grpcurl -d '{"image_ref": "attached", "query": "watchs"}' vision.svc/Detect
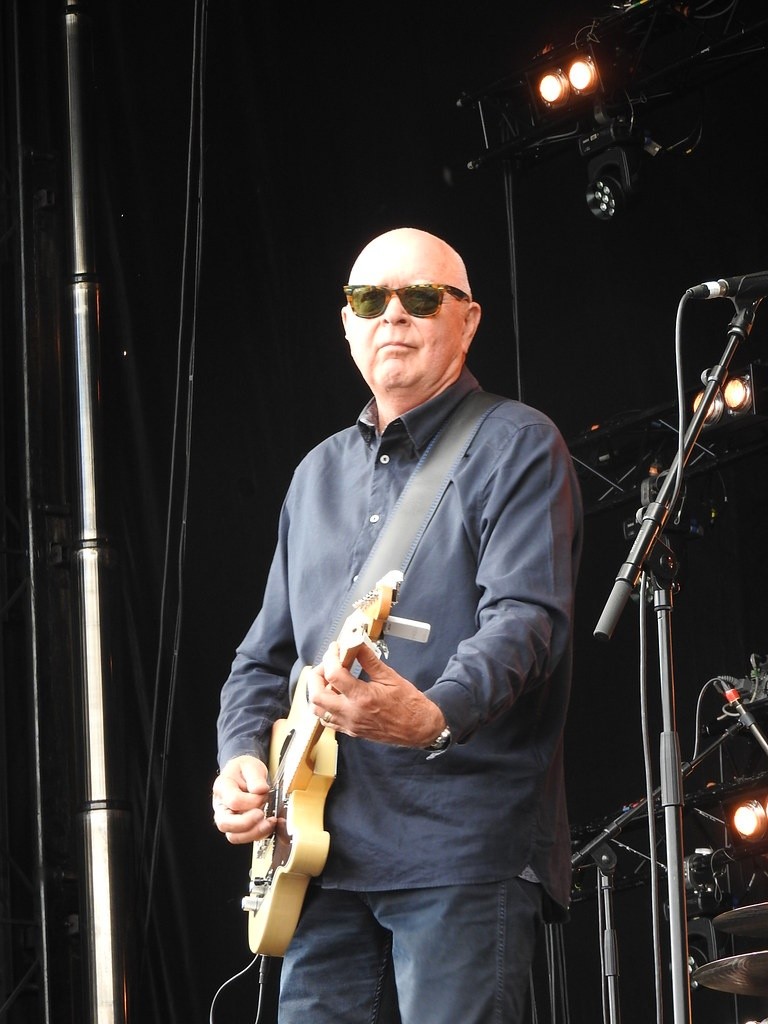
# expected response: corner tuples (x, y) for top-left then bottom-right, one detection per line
(424, 722), (453, 754)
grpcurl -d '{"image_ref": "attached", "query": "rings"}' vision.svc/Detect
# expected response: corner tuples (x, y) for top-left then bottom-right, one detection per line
(322, 710), (333, 723)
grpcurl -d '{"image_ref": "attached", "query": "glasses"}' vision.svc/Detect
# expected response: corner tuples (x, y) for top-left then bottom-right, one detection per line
(343, 285), (472, 319)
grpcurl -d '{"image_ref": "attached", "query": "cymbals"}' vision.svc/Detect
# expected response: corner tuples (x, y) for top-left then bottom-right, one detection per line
(710, 900), (768, 940)
(692, 950), (768, 1001)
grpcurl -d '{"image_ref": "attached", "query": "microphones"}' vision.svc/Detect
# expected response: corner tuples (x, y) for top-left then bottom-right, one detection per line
(686, 270), (768, 304)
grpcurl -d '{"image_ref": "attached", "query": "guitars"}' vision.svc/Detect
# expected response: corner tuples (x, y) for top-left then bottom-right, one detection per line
(238, 571), (403, 958)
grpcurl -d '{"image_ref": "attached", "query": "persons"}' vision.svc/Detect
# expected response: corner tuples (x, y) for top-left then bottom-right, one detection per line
(209, 227), (580, 1024)
